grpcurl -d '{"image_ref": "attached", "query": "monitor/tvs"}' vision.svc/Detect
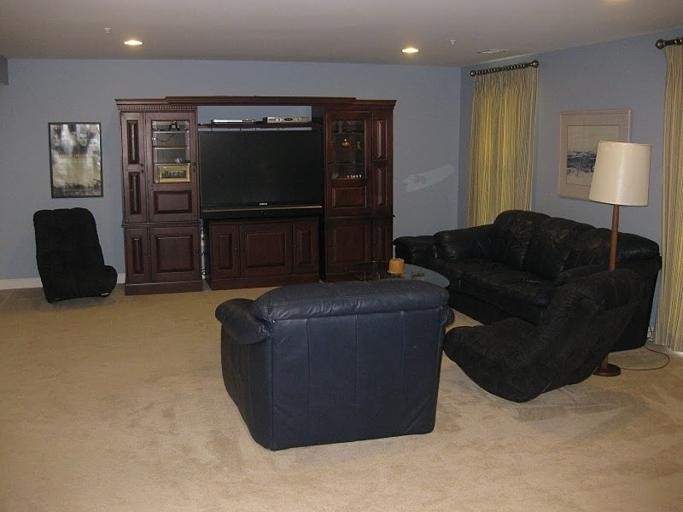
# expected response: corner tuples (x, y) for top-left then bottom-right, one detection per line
(198, 124), (324, 219)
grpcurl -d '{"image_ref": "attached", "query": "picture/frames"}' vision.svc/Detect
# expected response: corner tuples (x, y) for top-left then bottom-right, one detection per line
(556, 106), (634, 203)
(47, 120), (106, 200)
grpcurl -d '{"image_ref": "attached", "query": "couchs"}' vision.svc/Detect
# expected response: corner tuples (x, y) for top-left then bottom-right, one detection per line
(392, 207), (665, 354)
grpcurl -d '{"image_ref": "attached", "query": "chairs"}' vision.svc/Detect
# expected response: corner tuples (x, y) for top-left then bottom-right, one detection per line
(211, 276), (456, 454)
(32, 206), (119, 304)
(443, 264), (650, 403)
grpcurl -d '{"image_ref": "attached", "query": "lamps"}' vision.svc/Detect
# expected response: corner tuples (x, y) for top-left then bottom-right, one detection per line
(588, 139), (653, 378)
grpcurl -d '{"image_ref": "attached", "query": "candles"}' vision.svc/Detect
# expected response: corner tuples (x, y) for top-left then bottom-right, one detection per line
(387, 256), (405, 272)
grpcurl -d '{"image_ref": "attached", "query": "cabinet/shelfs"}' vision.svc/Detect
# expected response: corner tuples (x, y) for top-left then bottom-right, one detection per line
(203, 218), (323, 292)
(311, 96), (399, 282)
(112, 98), (202, 296)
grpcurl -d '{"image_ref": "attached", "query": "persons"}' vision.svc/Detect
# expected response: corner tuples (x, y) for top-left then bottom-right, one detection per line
(50, 123), (100, 164)
